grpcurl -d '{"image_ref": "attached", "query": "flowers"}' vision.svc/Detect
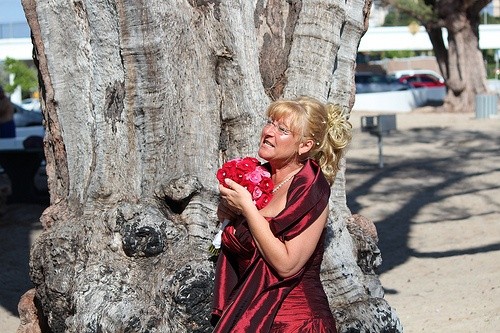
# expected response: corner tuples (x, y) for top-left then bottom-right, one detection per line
(207, 156), (274, 257)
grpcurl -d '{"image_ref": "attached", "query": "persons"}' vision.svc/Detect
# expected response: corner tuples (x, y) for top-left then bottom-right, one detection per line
(212, 96), (353, 333)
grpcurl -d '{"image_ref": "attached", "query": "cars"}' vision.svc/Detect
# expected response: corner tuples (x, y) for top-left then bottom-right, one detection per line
(21, 98), (41, 113)
(0, 99), (47, 149)
(399, 74), (447, 88)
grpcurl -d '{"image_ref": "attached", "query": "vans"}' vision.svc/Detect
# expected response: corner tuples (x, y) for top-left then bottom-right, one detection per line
(395, 69), (445, 84)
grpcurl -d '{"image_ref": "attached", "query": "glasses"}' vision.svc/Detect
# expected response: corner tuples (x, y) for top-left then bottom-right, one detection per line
(264, 118), (308, 138)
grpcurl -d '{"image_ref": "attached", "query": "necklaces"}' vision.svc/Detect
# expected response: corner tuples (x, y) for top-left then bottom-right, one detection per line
(272, 165), (304, 192)
